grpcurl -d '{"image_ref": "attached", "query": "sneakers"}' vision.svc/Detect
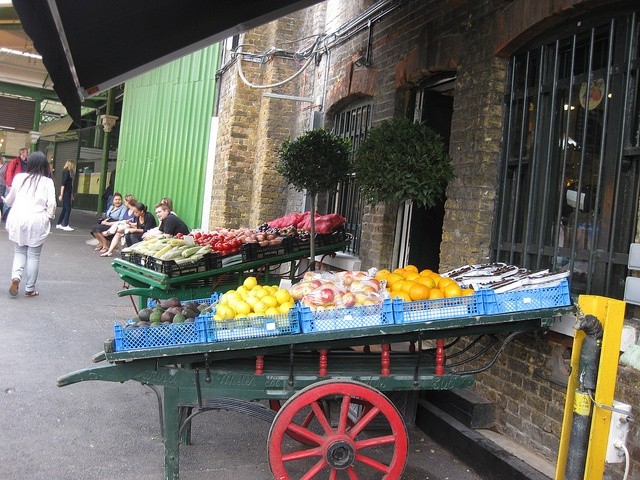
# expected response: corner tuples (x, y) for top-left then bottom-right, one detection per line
(24, 291), (39, 296)
(62, 226), (74, 231)
(9, 278), (20, 296)
(56, 224), (63, 229)
(101, 252), (112, 257)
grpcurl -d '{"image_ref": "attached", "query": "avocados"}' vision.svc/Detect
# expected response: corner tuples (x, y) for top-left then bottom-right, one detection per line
(127, 297), (209, 333)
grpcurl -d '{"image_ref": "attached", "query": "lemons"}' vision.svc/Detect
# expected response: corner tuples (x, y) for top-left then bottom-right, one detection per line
(213, 277), (294, 330)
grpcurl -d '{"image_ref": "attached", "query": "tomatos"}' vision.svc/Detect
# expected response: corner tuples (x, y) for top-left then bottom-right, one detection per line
(176, 231), (243, 257)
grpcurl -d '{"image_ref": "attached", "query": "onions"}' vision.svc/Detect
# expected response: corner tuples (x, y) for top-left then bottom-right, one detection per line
(254, 222), (317, 240)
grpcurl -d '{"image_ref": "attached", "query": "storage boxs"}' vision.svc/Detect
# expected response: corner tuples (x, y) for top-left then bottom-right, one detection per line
(484, 277), (571, 315)
(113, 293), (218, 351)
(205, 302), (300, 345)
(394, 290), (483, 326)
(301, 296), (393, 333)
(121, 221), (346, 277)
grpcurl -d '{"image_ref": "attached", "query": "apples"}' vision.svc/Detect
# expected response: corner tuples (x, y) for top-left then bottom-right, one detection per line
(293, 268), (388, 326)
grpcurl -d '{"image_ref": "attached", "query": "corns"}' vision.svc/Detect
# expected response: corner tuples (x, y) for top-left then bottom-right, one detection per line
(122, 234), (211, 272)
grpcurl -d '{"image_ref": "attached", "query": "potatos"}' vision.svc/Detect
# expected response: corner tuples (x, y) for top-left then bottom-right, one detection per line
(213, 228), (283, 246)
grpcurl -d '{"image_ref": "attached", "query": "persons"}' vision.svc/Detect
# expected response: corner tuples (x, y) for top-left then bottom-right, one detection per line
(156, 198), (177, 228)
(0, 153), (8, 213)
(102, 169), (115, 218)
(154, 203), (189, 235)
(100, 194), (138, 257)
(56, 159), (76, 231)
(90, 192), (128, 253)
(122, 203), (157, 247)
(0, 150), (57, 297)
(1, 148), (28, 224)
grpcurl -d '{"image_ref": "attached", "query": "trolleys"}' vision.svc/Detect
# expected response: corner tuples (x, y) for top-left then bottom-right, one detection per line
(285, 406), (289, 410)
(115, 229), (351, 301)
(58, 304), (579, 480)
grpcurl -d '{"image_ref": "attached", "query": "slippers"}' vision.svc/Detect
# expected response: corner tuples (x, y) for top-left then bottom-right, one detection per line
(100, 248), (109, 252)
(95, 245), (103, 250)
